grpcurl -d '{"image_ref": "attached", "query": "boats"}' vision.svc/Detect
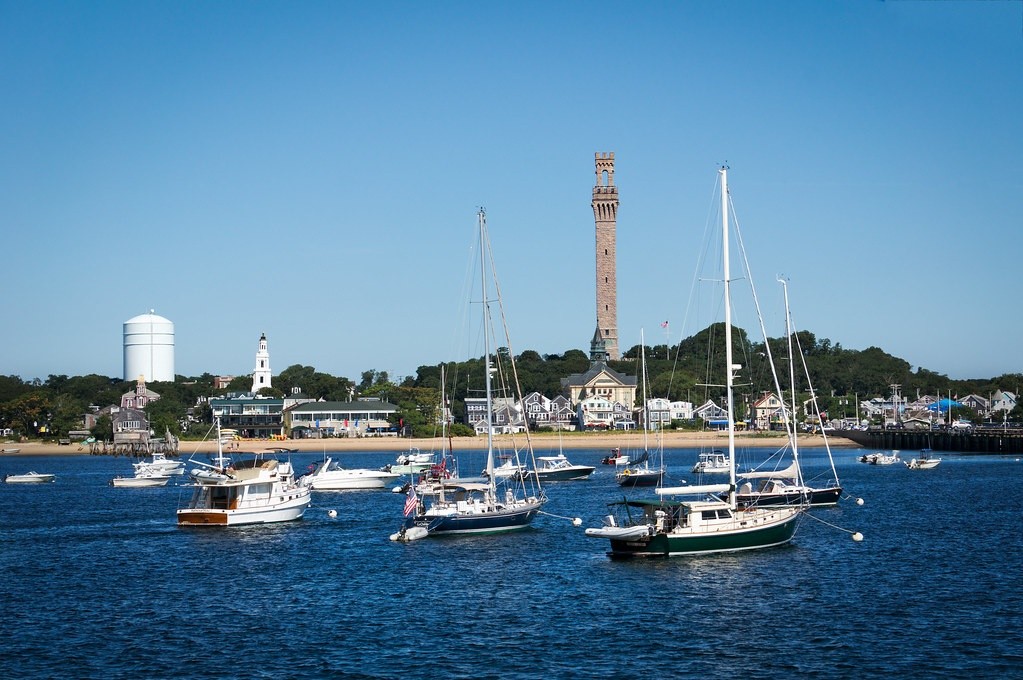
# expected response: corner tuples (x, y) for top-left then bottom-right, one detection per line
(295, 441), (402, 491)
(903, 435), (943, 471)
(132, 451), (187, 476)
(5, 471), (59, 484)
(1, 448), (22, 453)
(174, 415), (312, 530)
(108, 459), (172, 488)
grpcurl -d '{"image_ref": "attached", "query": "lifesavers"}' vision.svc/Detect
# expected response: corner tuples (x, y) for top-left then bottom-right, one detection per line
(624, 470), (629, 474)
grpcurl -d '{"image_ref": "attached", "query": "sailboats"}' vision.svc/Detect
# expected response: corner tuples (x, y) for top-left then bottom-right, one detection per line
(377, 158), (901, 562)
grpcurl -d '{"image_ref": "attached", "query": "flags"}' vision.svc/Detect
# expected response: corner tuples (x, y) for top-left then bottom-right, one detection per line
(404, 488), (418, 516)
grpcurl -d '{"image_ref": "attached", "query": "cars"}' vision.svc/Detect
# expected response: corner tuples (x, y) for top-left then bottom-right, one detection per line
(951, 420), (973, 431)
(0, 428), (13, 437)
(806, 425), (820, 433)
(819, 424), (835, 432)
(866, 422), (886, 435)
(886, 421), (904, 431)
